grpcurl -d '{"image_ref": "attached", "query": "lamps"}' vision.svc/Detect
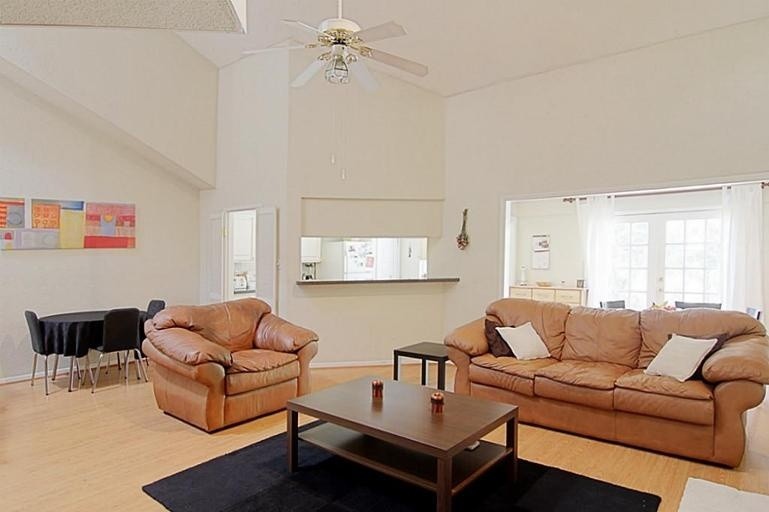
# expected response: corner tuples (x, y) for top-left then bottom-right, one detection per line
(322, 45), (357, 88)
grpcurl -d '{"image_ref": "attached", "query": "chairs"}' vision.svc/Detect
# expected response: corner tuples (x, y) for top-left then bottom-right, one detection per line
(23, 310), (94, 392)
(83, 307), (149, 393)
(598, 299), (762, 321)
(125, 300), (167, 383)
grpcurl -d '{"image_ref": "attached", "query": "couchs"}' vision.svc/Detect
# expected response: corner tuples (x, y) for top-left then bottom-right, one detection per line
(446, 298), (767, 477)
(142, 296), (319, 432)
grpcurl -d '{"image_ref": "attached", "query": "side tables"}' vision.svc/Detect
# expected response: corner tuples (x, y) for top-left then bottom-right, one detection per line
(392, 340), (449, 389)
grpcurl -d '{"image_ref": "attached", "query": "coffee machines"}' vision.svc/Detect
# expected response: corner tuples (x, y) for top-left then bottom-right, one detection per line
(301, 262), (317, 281)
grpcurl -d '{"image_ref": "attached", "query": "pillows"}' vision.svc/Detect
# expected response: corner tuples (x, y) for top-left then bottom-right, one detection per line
(483, 319), (511, 357)
(495, 321), (553, 362)
(667, 333), (728, 379)
(643, 332), (719, 385)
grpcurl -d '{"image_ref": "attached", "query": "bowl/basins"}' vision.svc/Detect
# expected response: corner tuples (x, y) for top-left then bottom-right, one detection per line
(537, 282), (552, 286)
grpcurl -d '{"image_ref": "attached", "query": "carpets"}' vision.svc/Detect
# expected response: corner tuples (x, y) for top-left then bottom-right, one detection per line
(677, 477), (768, 512)
(142, 421), (664, 512)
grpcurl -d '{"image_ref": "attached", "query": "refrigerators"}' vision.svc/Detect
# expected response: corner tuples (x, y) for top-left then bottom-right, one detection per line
(322, 237), (378, 281)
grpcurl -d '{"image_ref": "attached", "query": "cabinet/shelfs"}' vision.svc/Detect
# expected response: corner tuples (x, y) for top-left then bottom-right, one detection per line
(230, 211), (256, 265)
(300, 235), (322, 263)
(508, 286), (590, 305)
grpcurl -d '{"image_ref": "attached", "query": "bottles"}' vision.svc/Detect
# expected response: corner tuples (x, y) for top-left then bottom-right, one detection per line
(430, 390), (444, 414)
(371, 379), (384, 398)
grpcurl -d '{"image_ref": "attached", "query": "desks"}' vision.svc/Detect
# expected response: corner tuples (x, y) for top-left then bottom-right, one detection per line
(38, 309), (146, 393)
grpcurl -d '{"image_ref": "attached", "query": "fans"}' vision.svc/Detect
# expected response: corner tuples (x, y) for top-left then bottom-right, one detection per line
(243, 0), (430, 89)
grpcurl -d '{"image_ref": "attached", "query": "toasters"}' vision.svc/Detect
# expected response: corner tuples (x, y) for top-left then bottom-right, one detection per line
(234, 272), (249, 289)
